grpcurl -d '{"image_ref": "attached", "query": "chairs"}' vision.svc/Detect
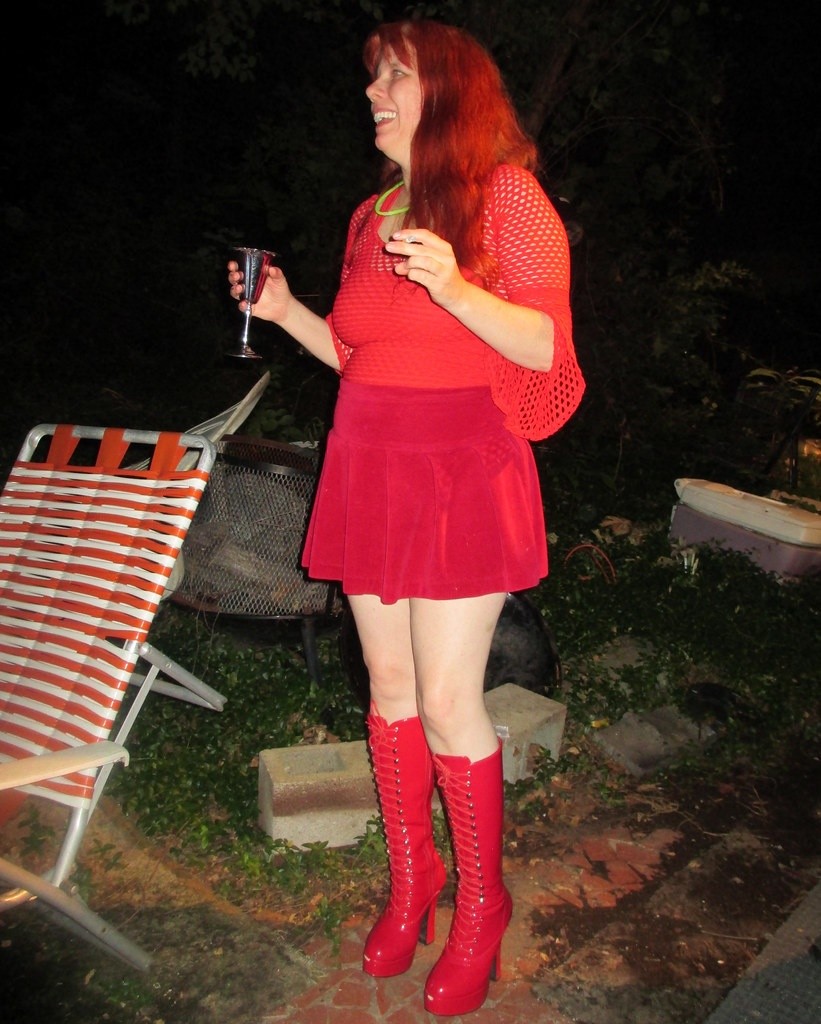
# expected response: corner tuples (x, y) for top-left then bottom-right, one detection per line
(0, 420), (227, 981)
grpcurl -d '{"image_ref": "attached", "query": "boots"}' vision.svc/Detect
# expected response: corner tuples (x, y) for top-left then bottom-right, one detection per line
(423, 735), (513, 1017)
(362, 714), (447, 977)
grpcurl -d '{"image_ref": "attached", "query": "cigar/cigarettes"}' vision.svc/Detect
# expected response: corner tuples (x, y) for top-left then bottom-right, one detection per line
(402, 237), (415, 244)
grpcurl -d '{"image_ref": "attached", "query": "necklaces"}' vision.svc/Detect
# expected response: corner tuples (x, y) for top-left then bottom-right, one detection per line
(374, 181), (428, 216)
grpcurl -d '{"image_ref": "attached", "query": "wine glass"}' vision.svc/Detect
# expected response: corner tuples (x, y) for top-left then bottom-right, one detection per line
(226, 246), (276, 360)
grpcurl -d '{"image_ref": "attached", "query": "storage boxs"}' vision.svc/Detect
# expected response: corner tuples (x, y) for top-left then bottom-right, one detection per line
(668, 478), (821, 584)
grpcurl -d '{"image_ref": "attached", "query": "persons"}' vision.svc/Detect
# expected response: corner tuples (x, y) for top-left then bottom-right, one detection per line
(229, 20), (585, 1017)
